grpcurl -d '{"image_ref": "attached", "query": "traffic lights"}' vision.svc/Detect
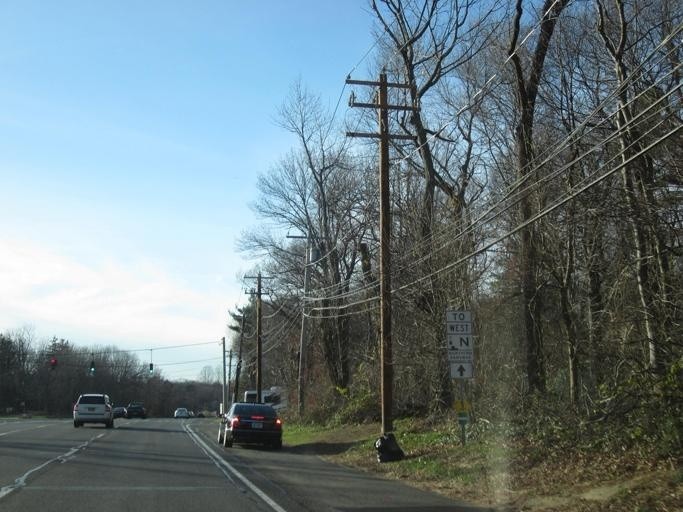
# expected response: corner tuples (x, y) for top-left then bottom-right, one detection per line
(49, 356), (56, 368)
(149, 363), (153, 374)
(90, 361), (95, 372)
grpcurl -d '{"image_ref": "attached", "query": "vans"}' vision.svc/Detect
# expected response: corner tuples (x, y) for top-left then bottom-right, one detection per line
(244, 389), (287, 410)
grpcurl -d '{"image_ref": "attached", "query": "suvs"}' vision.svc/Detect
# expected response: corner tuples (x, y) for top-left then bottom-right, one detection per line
(73, 393), (114, 427)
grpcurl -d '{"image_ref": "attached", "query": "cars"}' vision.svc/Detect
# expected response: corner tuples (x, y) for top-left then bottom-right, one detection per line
(174, 408), (189, 418)
(217, 402), (283, 451)
(128, 400), (146, 419)
(114, 406), (127, 418)
